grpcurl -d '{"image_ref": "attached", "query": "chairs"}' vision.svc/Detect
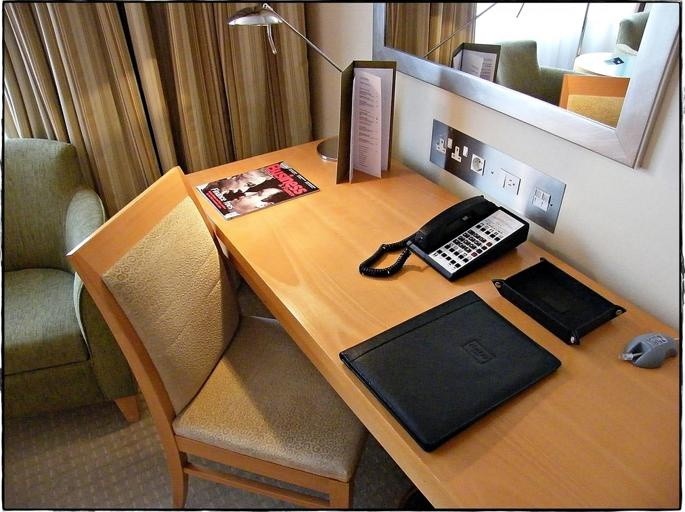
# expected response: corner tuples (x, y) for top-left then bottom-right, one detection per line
(494, 38), (538, 95)
(66, 166), (370, 511)
(3, 136), (142, 425)
(616, 14), (647, 50)
(563, 72), (624, 130)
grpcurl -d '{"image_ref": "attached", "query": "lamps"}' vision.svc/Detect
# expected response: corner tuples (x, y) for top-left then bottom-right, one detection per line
(228, 2), (358, 160)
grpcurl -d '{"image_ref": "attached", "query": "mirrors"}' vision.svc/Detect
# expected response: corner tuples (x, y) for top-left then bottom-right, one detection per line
(371, 0), (682, 168)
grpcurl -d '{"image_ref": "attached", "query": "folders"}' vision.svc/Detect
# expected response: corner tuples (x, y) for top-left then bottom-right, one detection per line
(339, 290), (562, 454)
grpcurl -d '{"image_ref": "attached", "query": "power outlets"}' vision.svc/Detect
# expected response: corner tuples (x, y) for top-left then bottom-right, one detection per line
(428, 118), (566, 234)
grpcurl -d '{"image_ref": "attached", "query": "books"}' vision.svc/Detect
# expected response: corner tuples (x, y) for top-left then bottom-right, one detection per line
(195, 160), (319, 222)
(337, 60), (396, 187)
(451, 42), (501, 84)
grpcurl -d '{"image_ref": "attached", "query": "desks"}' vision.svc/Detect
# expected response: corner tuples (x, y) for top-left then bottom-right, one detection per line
(574, 51), (638, 78)
(181, 131), (679, 508)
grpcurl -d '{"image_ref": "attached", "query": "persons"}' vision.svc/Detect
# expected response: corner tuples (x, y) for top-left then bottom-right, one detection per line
(223, 187), (288, 219)
(197, 167), (273, 195)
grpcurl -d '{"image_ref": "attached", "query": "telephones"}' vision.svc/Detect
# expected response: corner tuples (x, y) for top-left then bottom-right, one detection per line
(358, 195), (530, 281)
